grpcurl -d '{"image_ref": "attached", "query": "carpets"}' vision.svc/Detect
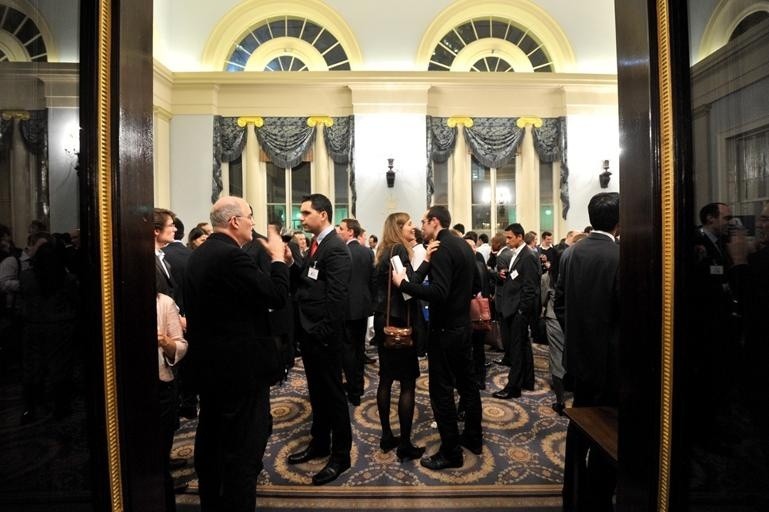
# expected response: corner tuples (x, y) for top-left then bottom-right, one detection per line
(165, 325), (575, 500)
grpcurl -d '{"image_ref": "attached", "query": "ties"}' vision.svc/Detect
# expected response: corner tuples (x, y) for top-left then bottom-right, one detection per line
(310, 238), (317, 256)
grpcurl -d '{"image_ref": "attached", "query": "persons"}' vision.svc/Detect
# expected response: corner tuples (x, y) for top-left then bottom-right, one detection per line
(551, 192), (619, 512)
(694, 201), (768, 320)
(185, 194), (291, 512)
(154, 191), (620, 496)
(0, 219), (80, 355)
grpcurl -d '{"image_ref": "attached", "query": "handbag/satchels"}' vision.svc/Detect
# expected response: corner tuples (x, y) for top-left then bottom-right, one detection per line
(471, 298), (493, 331)
(383, 326), (413, 348)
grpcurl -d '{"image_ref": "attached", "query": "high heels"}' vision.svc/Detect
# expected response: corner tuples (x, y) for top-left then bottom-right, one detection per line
(397, 447), (426, 462)
(380, 433), (400, 454)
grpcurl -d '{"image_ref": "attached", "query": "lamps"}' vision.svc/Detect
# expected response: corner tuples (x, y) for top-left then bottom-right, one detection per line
(598, 155), (614, 189)
(383, 157), (398, 189)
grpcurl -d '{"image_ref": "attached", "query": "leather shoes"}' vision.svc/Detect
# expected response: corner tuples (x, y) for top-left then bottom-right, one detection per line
(421, 453), (463, 470)
(288, 448), (317, 464)
(312, 466), (339, 485)
(492, 390), (522, 399)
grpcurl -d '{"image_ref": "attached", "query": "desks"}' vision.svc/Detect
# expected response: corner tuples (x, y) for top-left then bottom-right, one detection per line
(559, 402), (623, 472)
